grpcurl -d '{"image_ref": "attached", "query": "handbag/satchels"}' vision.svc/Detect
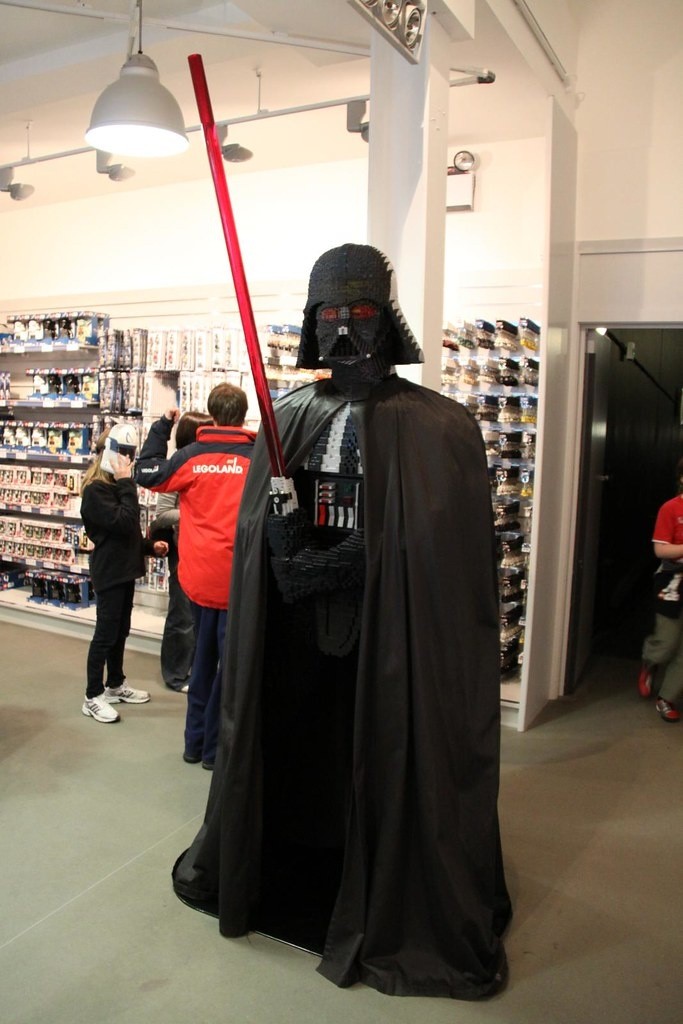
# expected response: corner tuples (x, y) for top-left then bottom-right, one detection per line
(652, 558), (683, 618)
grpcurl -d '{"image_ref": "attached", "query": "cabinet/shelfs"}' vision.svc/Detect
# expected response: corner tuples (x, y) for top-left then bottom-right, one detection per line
(103, 366), (259, 429)
(0, 310), (109, 612)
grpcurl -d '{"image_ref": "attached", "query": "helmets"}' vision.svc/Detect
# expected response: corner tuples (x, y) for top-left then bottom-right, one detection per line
(296, 243), (424, 370)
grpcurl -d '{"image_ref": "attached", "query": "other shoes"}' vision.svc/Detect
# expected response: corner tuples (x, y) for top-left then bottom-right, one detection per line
(202, 761), (215, 770)
(180, 685), (189, 693)
(183, 752), (202, 763)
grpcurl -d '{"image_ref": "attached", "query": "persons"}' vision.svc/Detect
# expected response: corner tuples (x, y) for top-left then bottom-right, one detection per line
(156, 411), (213, 693)
(136, 382), (259, 769)
(169, 243), (514, 1003)
(636, 460), (683, 722)
(79, 425), (171, 723)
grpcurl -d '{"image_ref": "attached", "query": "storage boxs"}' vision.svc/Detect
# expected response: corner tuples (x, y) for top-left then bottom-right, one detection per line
(134, 556), (170, 593)
(0, 569), (99, 611)
(0, 419), (93, 457)
(137, 485), (157, 538)
(0, 464), (87, 565)
(4, 311), (111, 346)
(0, 367), (101, 403)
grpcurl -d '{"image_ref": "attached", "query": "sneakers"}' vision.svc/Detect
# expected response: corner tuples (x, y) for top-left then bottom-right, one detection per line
(82, 694), (120, 722)
(655, 698), (681, 722)
(638, 663), (653, 698)
(103, 676), (150, 703)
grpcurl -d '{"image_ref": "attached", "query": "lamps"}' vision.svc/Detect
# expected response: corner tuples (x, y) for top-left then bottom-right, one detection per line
(446, 151), (476, 208)
(94, 150), (136, 184)
(0, 167), (36, 202)
(83, 0), (191, 159)
(347, 101), (369, 143)
(215, 125), (253, 163)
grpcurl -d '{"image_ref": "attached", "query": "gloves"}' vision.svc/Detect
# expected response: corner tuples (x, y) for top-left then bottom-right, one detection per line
(267, 508), (318, 557)
(271, 528), (364, 604)
